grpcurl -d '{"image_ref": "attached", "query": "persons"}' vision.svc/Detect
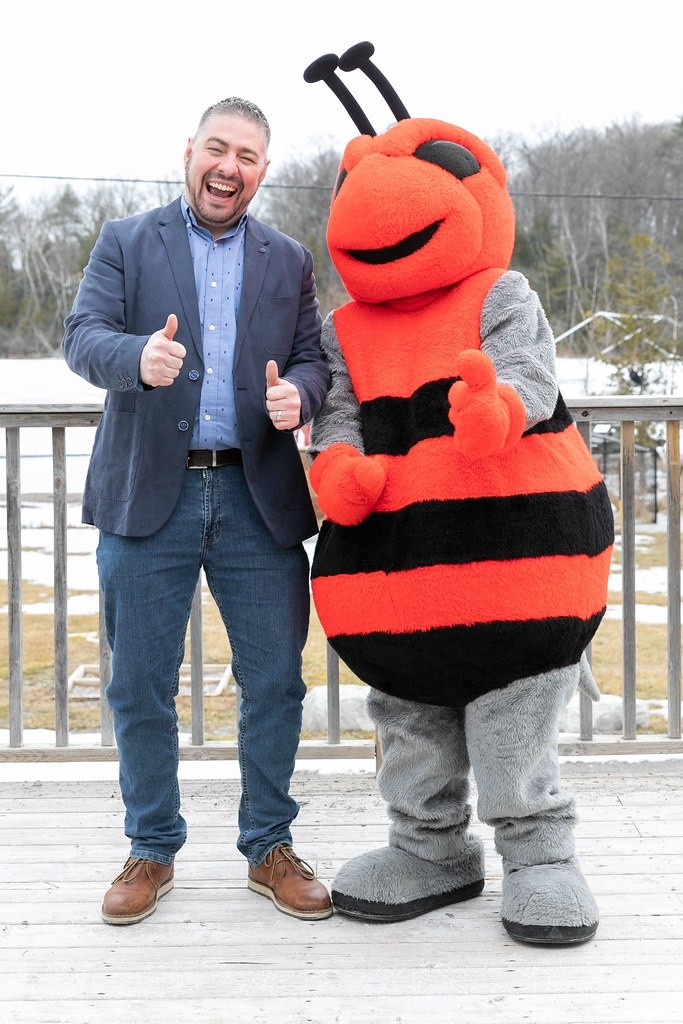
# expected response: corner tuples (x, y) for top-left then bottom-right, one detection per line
(61, 97), (347, 926)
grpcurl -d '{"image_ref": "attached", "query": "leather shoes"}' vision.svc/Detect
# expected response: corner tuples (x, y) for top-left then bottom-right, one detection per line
(247, 841), (334, 921)
(101, 855), (175, 926)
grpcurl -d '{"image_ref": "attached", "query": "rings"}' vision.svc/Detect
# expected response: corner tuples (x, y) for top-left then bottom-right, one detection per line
(277, 411), (282, 422)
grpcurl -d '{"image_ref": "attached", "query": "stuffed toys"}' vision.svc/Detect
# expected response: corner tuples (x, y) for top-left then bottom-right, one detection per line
(302, 41), (615, 945)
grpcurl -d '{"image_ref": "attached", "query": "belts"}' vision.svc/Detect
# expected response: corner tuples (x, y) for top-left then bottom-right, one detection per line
(186, 447), (242, 470)
